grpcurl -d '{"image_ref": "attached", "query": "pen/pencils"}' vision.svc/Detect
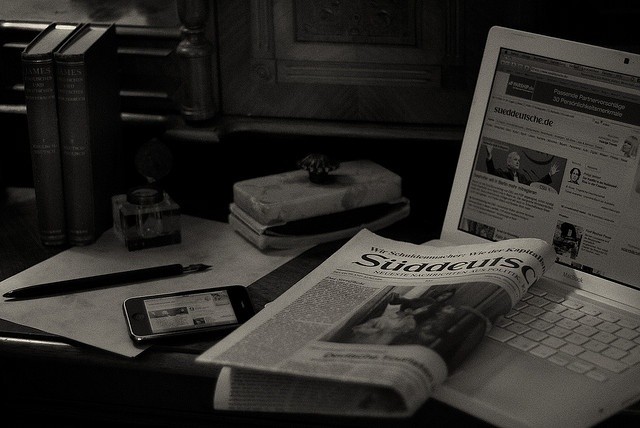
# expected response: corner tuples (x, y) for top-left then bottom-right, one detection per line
(3, 264), (213, 298)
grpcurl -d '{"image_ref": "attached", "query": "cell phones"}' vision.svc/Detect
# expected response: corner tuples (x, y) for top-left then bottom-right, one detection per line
(123, 284), (256, 344)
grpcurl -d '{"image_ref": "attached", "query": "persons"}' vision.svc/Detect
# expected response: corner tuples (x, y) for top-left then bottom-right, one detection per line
(620, 136), (638, 157)
(568, 168), (581, 186)
(350, 287), (456, 345)
(484, 140), (560, 186)
(150, 307), (184, 317)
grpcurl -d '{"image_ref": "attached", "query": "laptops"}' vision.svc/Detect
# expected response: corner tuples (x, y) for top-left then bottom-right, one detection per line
(419, 24), (639, 427)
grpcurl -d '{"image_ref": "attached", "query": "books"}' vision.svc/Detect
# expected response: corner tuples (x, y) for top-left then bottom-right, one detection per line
(21, 22), (82, 248)
(53, 22), (129, 247)
